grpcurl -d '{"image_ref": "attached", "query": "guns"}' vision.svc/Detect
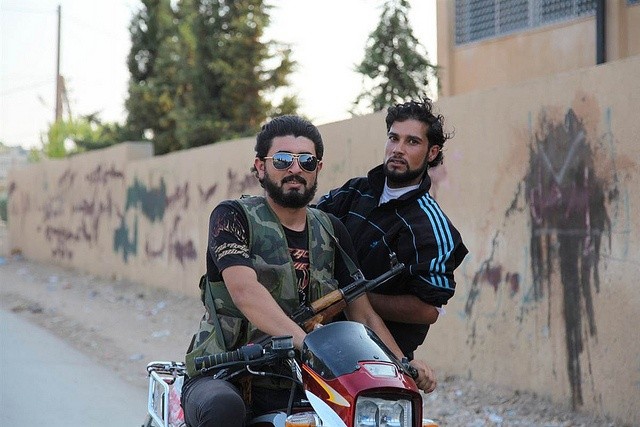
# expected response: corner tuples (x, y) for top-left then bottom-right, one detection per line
(213, 261), (405, 378)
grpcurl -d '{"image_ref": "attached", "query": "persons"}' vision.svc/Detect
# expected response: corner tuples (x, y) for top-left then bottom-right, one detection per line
(181, 116), (436, 427)
(309, 100), (468, 364)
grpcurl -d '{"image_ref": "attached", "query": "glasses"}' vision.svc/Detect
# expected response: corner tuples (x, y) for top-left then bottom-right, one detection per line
(264, 153), (320, 173)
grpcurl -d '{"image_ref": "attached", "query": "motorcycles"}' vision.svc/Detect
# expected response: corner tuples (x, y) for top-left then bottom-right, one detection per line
(142, 320), (437, 426)
